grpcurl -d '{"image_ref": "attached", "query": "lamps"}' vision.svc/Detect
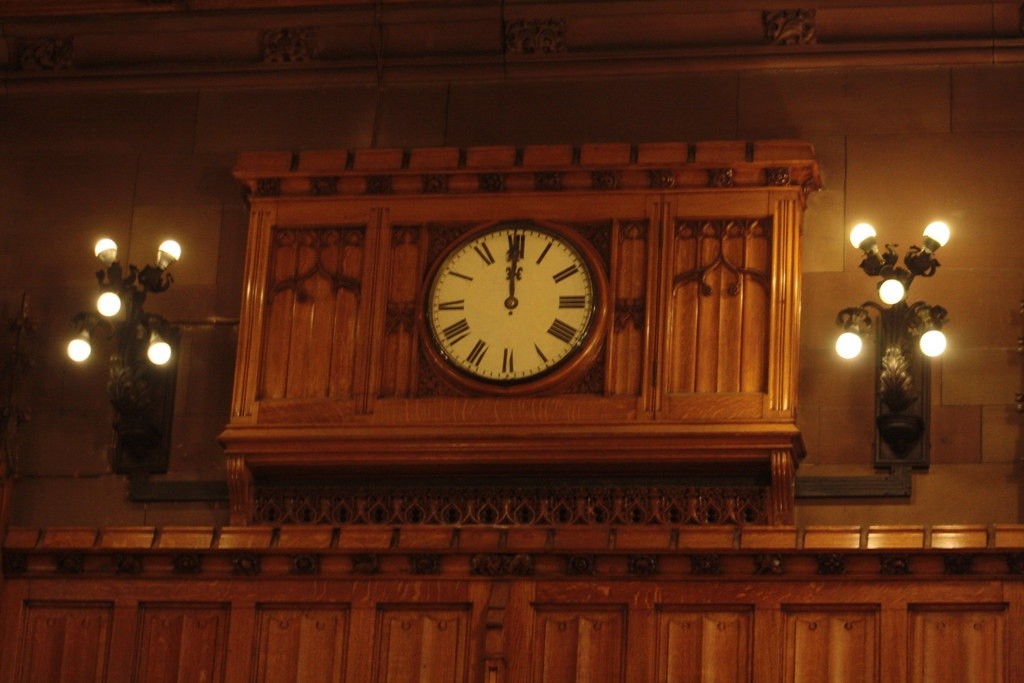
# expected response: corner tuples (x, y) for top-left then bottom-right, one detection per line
(62, 234), (188, 476)
(827, 216), (957, 474)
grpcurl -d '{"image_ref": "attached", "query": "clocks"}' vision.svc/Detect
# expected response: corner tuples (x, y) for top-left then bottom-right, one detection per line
(425, 221), (610, 396)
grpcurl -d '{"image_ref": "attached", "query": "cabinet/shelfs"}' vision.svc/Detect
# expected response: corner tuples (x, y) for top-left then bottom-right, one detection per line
(216, 137), (834, 527)
